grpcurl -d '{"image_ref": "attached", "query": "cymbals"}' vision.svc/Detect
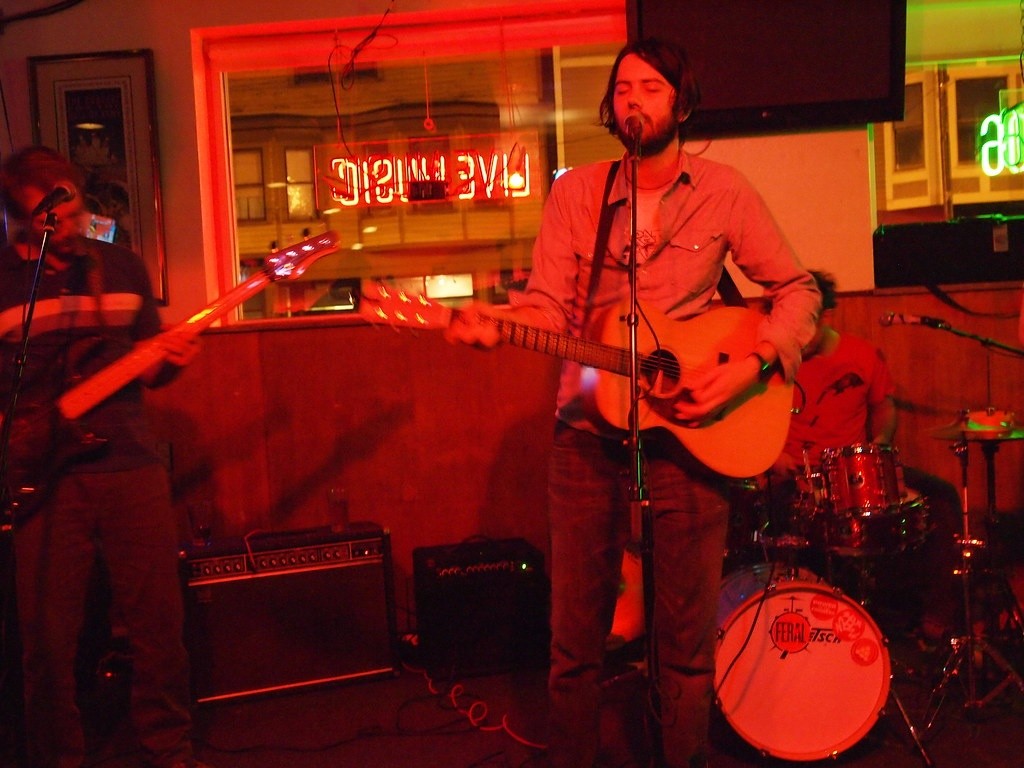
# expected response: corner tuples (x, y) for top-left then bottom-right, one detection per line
(931, 404), (1024, 446)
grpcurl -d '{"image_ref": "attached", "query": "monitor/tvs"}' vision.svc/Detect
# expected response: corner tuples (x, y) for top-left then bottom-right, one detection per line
(625, 0), (905, 140)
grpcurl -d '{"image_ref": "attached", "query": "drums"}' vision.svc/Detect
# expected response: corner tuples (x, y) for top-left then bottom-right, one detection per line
(812, 482), (936, 561)
(713, 561), (895, 766)
(728, 480), (814, 562)
(821, 442), (902, 517)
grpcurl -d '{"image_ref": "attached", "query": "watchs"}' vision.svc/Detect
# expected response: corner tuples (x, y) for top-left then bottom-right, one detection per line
(760, 358), (770, 378)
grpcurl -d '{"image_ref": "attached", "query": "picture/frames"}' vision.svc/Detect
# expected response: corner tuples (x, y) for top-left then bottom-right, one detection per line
(27, 46), (169, 307)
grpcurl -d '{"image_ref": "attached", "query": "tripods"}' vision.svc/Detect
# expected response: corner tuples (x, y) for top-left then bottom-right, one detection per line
(812, 508), (938, 768)
(927, 436), (1024, 717)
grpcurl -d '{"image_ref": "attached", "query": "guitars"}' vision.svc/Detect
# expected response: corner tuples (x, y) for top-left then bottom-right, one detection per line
(0, 226), (343, 548)
(354, 272), (795, 479)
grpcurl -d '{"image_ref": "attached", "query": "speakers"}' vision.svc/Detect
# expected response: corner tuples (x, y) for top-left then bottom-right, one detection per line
(873, 221), (979, 286)
(959, 202), (1022, 282)
(412, 535), (548, 684)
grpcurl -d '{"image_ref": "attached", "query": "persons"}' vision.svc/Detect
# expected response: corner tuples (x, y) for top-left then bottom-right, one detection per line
(0, 143), (202, 768)
(461, 37), (826, 768)
(89, 181), (132, 250)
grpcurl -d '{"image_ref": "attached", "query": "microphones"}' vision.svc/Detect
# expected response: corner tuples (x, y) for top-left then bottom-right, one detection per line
(624, 117), (643, 145)
(32, 181), (76, 213)
(878, 310), (947, 326)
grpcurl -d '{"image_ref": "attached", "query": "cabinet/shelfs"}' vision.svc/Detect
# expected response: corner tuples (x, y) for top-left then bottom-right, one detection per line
(868, 57), (1024, 206)
